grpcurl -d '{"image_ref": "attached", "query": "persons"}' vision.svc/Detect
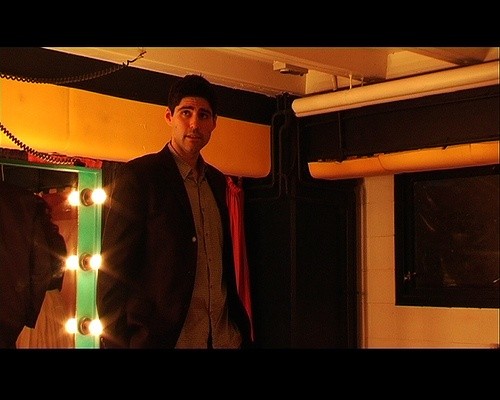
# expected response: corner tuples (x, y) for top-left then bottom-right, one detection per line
(94, 73), (256, 350)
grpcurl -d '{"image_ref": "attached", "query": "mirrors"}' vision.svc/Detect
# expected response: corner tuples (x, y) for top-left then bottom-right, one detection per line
(1, 165), (80, 349)
